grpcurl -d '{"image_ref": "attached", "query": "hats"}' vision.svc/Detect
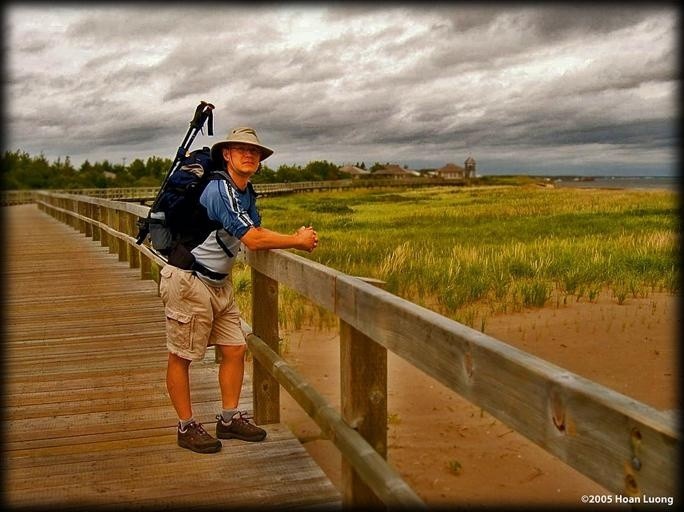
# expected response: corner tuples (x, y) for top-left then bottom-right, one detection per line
(210, 127), (274, 170)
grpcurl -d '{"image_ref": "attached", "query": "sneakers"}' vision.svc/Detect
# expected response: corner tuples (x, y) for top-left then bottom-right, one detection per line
(215, 411), (266, 441)
(178, 421), (222, 453)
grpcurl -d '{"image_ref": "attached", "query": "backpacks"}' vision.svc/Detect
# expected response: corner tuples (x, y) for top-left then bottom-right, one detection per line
(148, 148), (237, 255)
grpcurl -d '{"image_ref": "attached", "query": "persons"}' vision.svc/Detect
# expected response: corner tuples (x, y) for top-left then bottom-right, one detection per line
(158, 127), (320, 454)
